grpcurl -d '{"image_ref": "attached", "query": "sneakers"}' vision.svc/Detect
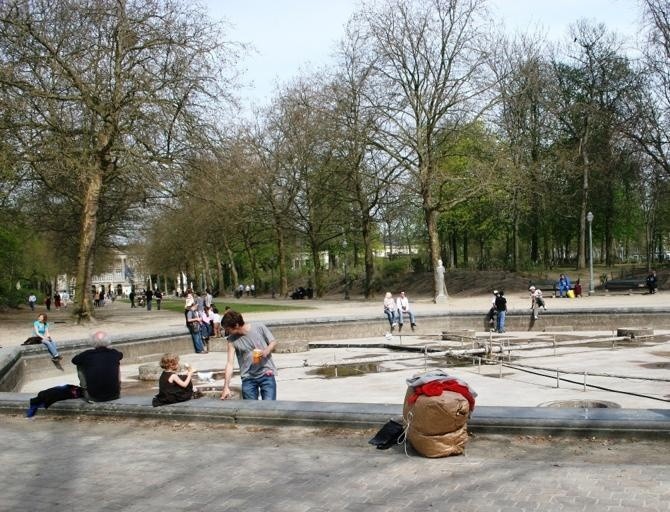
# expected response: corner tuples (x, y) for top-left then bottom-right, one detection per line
(489, 319), (493, 323)
(534, 316), (538, 319)
(194, 390), (206, 399)
(412, 323), (416, 326)
(198, 351), (208, 354)
(391, 322), (403, 327)
(52, 356), (63, 361)
(544, 306), (547, 310)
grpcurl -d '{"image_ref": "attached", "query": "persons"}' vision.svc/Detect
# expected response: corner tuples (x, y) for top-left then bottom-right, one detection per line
(529, 285), (547, 320)
(396, 290), (416, 327)
(559, 273), (570, 298)
(489, 290), (499, 324)
(151, 352), (206, 408)
(495, 290), (508, 334)
(575, 279), (582, 299)
(71, 331), (124, 402)
(219, 310), (278, 401)
(28, 283), (255, 362)
(383, 291), (397, 327)
(645, 270), (657, 294)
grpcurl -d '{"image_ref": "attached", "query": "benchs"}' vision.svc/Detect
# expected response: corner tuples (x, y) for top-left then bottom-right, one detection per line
(528, 279), (579, 297)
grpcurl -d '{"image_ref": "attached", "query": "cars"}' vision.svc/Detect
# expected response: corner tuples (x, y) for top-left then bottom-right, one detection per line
(634, 250), (670, 261)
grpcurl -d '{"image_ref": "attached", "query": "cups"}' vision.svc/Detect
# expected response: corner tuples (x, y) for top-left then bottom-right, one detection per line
(254, 349), (262, 365)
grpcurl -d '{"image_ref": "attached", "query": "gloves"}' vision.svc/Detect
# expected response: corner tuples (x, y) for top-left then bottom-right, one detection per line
(368, 416), (405, 449)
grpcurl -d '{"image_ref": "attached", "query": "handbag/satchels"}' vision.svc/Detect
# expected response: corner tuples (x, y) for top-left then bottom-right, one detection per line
(24, 337), (42, 344)
(567, 290), (573, 298)
(403, 372), (469, 457)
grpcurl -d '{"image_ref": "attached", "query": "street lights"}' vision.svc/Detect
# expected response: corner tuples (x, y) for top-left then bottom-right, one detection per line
(342, 261), (350, 299)
(586, 210), (599, 296)
(228, 263), (234, 295)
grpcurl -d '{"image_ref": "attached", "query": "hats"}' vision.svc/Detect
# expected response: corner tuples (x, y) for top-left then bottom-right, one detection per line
(494, 290), (498, 294)
(530, 286), (535, 290)
(210, 304), (216, 307)
